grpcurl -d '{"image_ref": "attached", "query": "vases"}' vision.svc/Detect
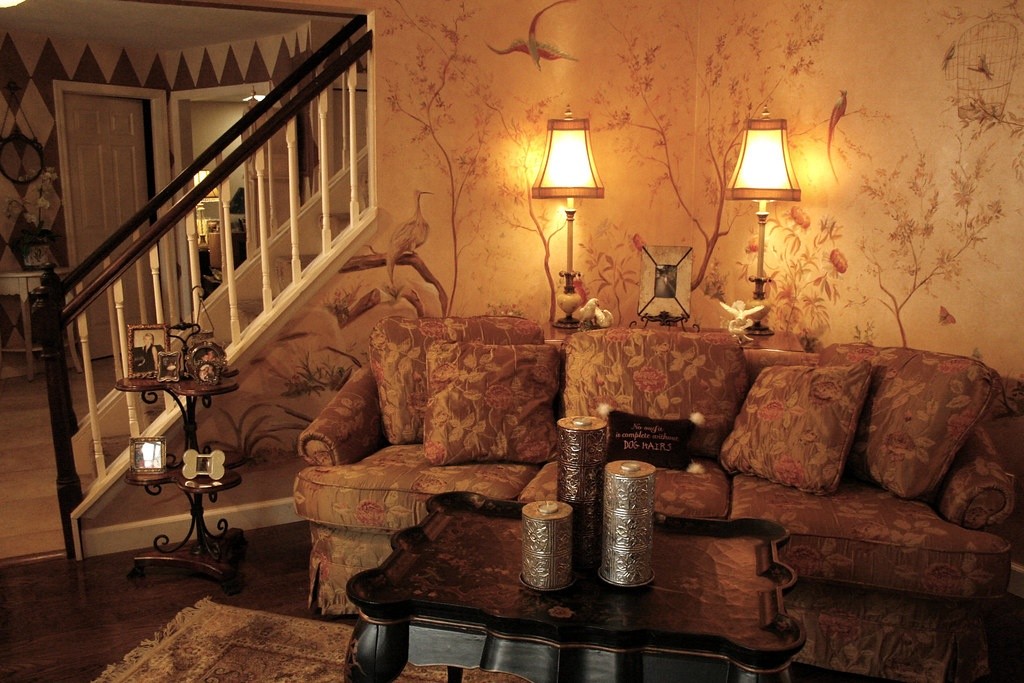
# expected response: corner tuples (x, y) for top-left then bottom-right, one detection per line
(24, 237), (60, 267)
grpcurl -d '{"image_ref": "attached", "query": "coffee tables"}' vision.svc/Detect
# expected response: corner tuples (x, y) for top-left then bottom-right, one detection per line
(343, 491), (807, 683)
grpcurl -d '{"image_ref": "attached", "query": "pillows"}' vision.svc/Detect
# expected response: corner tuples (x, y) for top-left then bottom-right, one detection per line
(814, 343), (1003, 500)
(563, 327), (749, 459)
(372, 313), (544, 445)
(597, 403), (707, 474)
(425, 341), (560, 466)
(720, 358), (874, 497)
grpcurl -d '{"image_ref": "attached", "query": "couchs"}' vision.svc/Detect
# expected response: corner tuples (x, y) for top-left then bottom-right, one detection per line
(295, 327), (1024, 683)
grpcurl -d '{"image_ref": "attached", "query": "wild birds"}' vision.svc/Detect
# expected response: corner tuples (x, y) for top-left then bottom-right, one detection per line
(719, 300), (764, 343)
(571, 297), (599, 330)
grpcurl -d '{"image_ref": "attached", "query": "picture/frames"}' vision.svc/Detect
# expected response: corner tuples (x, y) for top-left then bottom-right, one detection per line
(128, 437), (167, 475)
(193, 359), (222, 386)
(182, 449), (225, 480)
(157, 350), (181, 383)
(185, 339), (228, 379)
(637, 246), (692, 318)
(127, 324), (171, 379)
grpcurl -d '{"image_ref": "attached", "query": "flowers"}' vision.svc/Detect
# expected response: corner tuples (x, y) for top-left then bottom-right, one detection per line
(0, 165), (63, 257)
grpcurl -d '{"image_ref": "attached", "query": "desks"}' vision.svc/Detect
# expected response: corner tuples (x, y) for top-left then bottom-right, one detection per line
(0, 266), (83, 381)
(540, 322), (808, 352)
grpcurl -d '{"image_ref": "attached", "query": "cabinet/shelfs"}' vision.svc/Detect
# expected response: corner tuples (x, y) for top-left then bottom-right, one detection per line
(116, 366), (249, 595)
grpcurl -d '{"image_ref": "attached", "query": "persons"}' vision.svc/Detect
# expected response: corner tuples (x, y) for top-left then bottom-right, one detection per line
(136, 334), (159, 373)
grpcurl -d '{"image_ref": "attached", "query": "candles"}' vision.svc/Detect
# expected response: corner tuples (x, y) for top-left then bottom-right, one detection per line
(573, 417), (591, 426)
(538, 500), (557, 513)
(621, 462), (640, 471)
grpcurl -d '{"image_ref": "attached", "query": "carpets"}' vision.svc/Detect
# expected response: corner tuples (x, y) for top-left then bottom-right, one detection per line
(87, 595), (532, 683)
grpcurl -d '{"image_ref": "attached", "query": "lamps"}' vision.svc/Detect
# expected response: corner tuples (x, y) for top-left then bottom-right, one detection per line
(230, 187), (245, 215)
(531, 105), (605, 327)
(726, 105), (802, 335)
(190, 285), (217, 346)
(194, 171), (220, 250)
